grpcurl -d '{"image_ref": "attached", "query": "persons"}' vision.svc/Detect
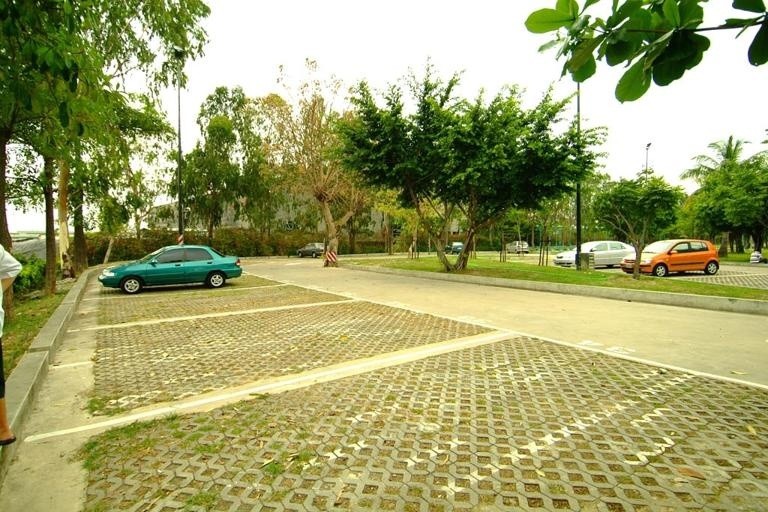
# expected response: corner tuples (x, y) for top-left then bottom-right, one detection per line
(61, 254), (75, 279)
(748, 248), (764, 264)
(0, 242), (23, 445)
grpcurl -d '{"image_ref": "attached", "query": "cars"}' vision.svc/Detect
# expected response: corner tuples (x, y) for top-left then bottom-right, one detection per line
(620, 239), (720, 277)
(506, 241), (529, 254)
(445, 241), (464, 255)
(296, 242), (324, 258)
(98, 244), (242, 294)
(553, 240), (636, 268)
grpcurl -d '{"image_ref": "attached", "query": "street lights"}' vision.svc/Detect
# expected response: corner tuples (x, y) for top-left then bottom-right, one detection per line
(646, 142), (653, 182)
